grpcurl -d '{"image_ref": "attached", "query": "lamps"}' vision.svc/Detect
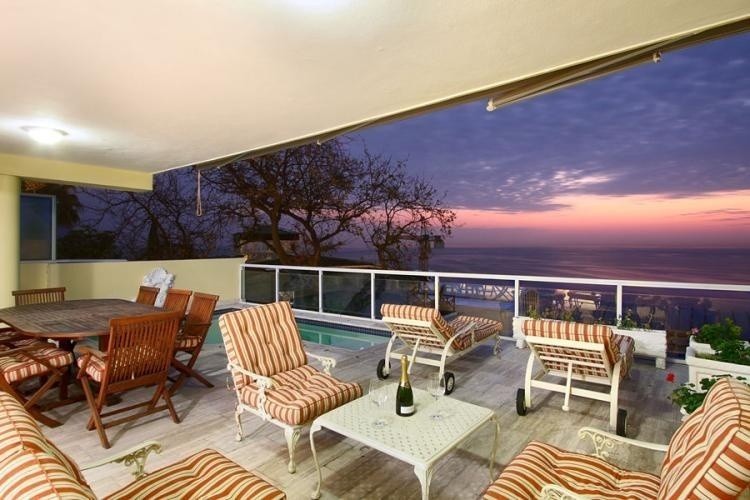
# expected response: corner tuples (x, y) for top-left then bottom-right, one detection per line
(21, 126), (68, 145)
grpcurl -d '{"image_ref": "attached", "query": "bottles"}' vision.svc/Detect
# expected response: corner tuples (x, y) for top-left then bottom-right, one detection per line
(395, 355), (415, 417)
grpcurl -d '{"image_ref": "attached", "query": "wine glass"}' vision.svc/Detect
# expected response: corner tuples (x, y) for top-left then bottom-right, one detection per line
(369, 377), (388, 429)
(427, 373), (446, 420)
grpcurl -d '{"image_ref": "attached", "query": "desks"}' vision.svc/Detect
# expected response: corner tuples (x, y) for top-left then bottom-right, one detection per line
(0, 299), (165, 406)
(309, 382), (501, 500)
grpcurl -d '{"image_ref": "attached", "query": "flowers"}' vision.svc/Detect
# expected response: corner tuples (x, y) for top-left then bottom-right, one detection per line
(665, 373), (750, 415)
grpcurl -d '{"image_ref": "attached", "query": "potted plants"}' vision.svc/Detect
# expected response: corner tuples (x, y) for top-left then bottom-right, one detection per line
(512, 304), (668, 370)
(665, 318), (750, 421)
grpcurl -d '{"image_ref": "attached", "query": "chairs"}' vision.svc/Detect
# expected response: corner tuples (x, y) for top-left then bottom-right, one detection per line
(136, 286), (160, 306)
(131, 266), (176, 308)
(0, 390), (286, 500)
(376, 303), (503, 395)
(12, 287), (65, 306)
(480, 376), (750, 500)
(76, 312), (186, 449)
(162, 292), (219, 400)
(163, 287), (193, 313)
(0, 318), (74, 428)
(516, 320), (635, 437)
(219, 301), (363, 474)
